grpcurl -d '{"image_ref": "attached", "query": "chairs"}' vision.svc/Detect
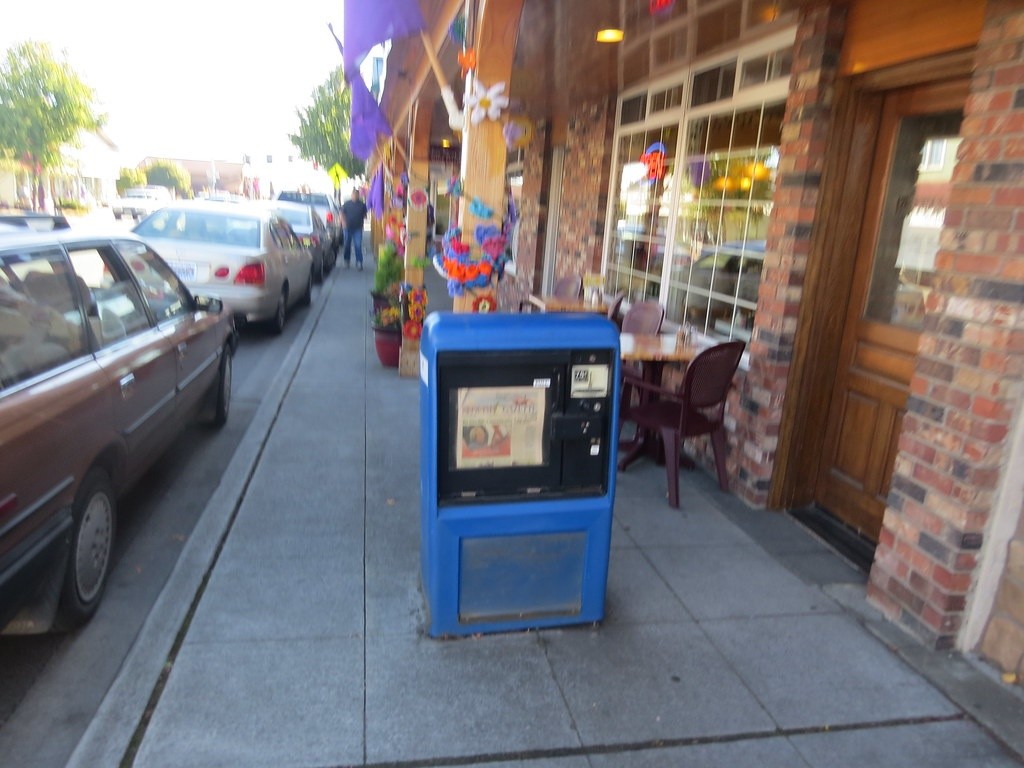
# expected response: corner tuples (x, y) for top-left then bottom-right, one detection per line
(553, 272), (584, 298)
(619, 297), (670, 412)
(602, 286), (628, 320)
(620, 339), (751, 511)
(28, 267), (106, 348)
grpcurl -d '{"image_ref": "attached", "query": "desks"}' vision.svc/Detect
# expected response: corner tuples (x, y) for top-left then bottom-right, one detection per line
(528, 293), (608, 314)
(617, 322), (718, 473)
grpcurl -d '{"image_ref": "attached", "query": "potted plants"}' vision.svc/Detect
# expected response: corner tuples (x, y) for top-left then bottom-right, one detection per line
(370, 243), (404, 314)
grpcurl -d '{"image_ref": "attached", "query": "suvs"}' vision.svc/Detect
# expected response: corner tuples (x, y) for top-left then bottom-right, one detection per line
(277, 189), (343, 252)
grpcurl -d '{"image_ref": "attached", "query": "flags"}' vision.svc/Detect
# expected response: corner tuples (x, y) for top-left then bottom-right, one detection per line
(366, 163), (384, 219)
(338, 43), (393, 163)
(339, 0), (425, 85)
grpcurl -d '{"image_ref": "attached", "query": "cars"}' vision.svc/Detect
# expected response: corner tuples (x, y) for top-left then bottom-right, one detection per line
(235, 200), (335, 287)
(0, 228), (239, 634)
(100, 199), (314, 336)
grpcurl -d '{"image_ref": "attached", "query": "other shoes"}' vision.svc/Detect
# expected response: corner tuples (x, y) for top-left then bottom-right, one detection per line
(355, 261), (364, 271)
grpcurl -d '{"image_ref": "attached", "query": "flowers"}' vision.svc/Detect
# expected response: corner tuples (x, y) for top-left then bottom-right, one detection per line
(373, 302), (401, 327)
(402, 283), (429, 340)
(462, 73), (512, 127)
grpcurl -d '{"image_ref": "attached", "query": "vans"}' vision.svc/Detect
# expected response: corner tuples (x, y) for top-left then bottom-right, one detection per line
(111, 185), (173, 224)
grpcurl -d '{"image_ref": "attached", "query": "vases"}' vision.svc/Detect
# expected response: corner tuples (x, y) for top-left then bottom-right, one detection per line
(373, 325), (401, 368)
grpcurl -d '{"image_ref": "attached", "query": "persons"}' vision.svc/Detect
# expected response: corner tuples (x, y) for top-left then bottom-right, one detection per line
(243, 177), (260, 199)
(37, 181), (45, 211)
(339, 189), (367, 270)
